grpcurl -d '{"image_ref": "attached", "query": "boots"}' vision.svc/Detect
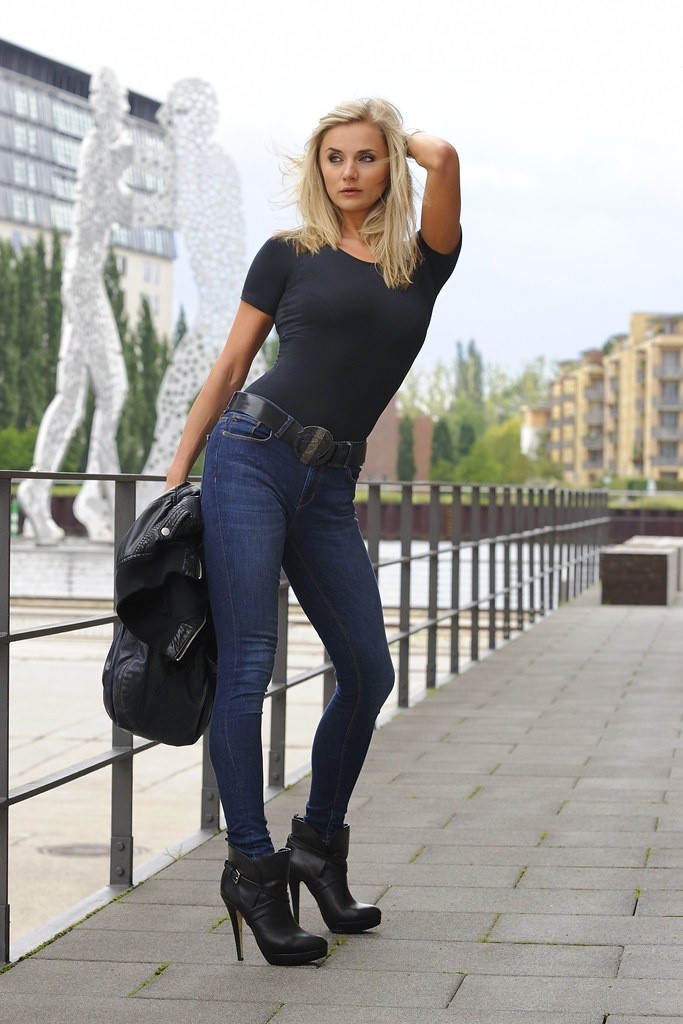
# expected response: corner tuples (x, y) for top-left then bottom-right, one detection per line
(219, 846), (327, 965)
(283, 815), (382, 932)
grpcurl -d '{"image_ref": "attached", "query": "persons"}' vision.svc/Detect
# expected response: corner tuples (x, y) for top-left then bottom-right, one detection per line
(14, 66), (267, 548)
(163, 97), (463, 966)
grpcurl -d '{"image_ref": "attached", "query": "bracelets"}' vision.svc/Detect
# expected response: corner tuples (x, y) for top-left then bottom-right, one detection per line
(405, 127), (423, 136)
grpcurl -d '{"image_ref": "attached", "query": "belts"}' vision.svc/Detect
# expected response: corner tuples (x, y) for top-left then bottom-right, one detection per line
(227, 392), (366, 471)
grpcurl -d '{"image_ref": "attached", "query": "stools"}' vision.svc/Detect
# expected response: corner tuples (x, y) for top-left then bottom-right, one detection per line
(624, 537), (683, 591)
(600, 544), (679, 605)
